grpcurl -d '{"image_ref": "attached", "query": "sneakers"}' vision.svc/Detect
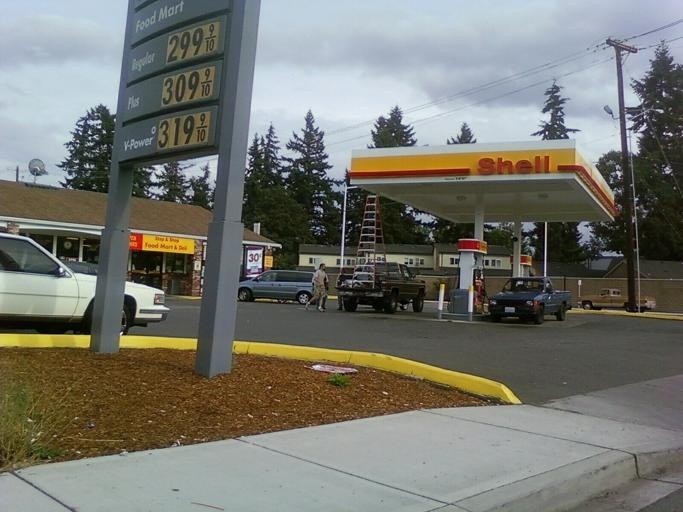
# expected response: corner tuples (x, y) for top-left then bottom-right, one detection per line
(305, 303), (308, 309)
(317, 306), (327, 312)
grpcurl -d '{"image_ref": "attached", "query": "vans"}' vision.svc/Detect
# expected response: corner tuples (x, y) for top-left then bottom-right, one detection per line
(238, 269), (318, 304)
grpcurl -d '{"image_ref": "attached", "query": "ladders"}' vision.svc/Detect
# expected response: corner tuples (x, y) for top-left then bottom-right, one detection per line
(353, 196), (388, 288)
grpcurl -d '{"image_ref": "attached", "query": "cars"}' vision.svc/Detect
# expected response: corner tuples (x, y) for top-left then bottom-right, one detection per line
(0, 232), (171, 336)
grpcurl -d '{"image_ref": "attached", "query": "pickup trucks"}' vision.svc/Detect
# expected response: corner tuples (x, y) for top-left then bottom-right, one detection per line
(488, 275), (573, 324)
(335, 262), (426, 315)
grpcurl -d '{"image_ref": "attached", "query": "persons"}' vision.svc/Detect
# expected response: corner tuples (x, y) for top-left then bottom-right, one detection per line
(303, 263), (329, 313)
(334, 266), (347, 311)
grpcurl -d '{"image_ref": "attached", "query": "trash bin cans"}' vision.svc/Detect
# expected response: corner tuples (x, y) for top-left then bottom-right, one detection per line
(450, 290), (469, 314)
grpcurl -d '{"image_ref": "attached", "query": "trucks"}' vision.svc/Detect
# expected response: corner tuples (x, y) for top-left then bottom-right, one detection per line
(579, 287), (657, 311)
